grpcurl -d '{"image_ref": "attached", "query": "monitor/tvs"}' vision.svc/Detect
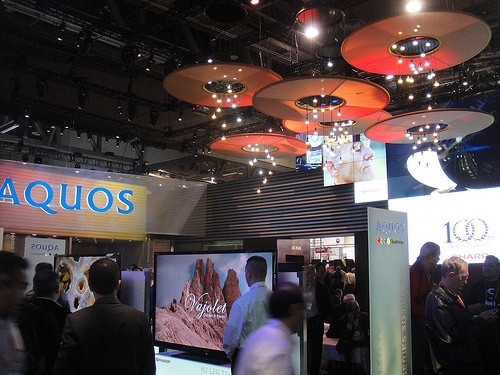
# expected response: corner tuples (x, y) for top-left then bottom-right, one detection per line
(152, 249), (277, 366)
(54, 252), (121, 313)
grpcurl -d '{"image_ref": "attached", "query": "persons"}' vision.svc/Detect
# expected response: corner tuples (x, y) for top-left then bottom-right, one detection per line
(312, 259), (369, 323)
(334, 300), (369, 370)
(325, 135), (375, 185)
(0, 250), (28, 375)
(425, 256), (497, 375)
(469, 255), (500, 375)
(26, 262), (71, 315)
(326, 294), (355, 338)
(19, 269), (67, 375)
(235, 282), (305, 375)
(222, 256), (272, 375)
(303, 265), (333, 375)
(51, 258), (156, 375)
(410, 242), (440, 375)
